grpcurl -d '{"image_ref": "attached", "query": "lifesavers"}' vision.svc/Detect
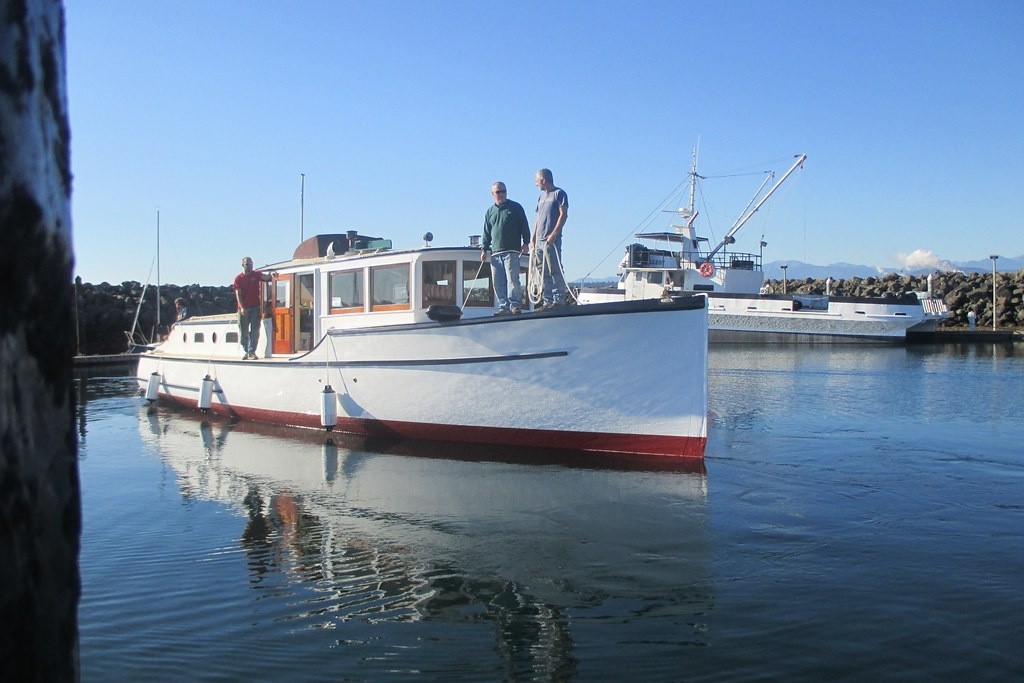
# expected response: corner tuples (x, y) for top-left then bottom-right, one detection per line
(700, 262), (714, 277)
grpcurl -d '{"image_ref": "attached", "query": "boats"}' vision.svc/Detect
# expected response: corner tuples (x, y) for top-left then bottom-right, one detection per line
(576, 134), (927, 338)
(137, 231), (709, 460)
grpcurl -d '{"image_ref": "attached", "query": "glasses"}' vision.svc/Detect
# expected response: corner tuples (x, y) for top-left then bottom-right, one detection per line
(494, 190), (507, 194)
(244, 262), (254, 265)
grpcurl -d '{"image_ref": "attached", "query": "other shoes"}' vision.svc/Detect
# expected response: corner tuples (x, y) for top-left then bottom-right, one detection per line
(243, 354), (258, 360)
(494, 305), (562, 316)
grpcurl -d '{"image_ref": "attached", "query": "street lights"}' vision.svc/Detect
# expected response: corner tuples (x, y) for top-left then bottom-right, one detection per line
(781, 265), (788, 294)
(990, 256), (998, 327)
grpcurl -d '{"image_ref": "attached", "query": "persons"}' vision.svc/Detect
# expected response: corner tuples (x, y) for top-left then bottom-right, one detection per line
(527, 168), (569, 313)
(232, 257), (279, 359)
(171, 297), (187, 330)
(480, 182), (530, 315)
